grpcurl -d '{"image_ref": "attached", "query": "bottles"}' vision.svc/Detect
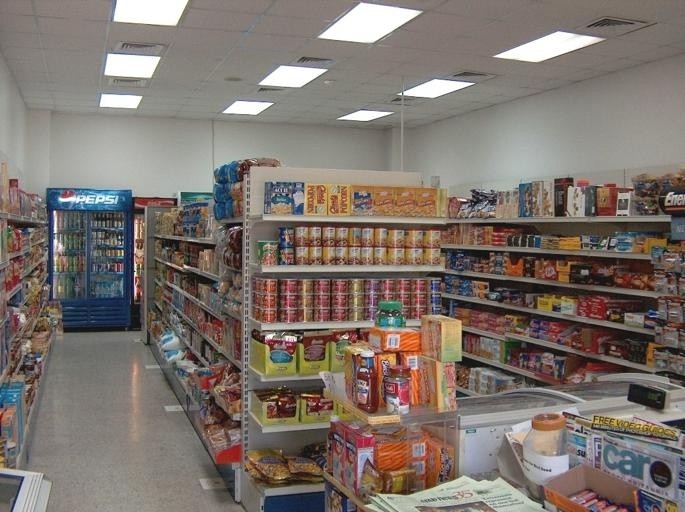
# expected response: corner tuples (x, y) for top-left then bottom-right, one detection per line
(56, 231), (124, 251)
(521, 411), (579, 503)
(56, 211), (124, 229)
(133, 215), (144, 303)
(355, 351), (378, 412)
(56, 273), (122, 298)
(53, 251), (84, 272)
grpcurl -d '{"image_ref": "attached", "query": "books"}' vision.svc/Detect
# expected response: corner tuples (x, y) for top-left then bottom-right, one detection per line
(363, 475), (546, 512)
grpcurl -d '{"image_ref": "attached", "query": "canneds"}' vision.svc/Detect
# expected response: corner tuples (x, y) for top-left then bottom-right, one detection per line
(389, 366), (411, 378)
(25, 361), (35, 376)
(92, 249), (124, 272)
(252, 226), (442, 328)
(385, 377), (409, 416)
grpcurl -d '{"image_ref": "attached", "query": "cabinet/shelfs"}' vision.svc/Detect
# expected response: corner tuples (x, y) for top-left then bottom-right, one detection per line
(441, 168), (685, 397)
(142, 166), (448, 512)
(0, 211), (57, 470)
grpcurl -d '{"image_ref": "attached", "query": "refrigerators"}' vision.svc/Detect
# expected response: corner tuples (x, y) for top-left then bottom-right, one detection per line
(44, 187), (132, 331)
(132, 196), (178, 329)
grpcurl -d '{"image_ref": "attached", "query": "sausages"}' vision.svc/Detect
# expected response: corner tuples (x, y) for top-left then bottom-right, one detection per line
(568, 489), (628, 512)
